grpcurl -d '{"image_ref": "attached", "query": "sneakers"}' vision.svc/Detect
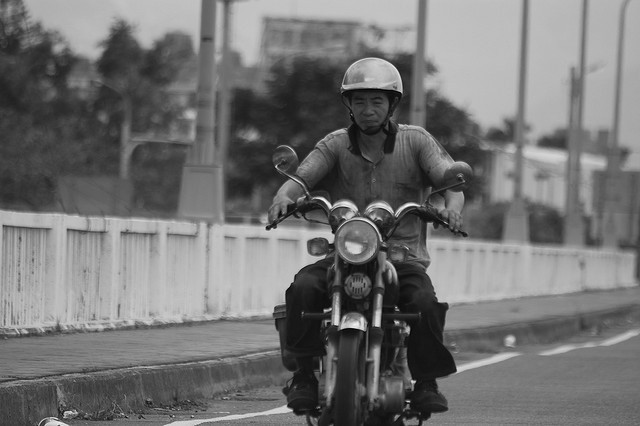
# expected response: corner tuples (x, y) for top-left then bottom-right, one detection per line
(288, 370), (319, 410)
(410, 386), (448, 414)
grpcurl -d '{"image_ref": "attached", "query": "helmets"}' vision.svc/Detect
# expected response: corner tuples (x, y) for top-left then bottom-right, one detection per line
(340, 57), (404, 100)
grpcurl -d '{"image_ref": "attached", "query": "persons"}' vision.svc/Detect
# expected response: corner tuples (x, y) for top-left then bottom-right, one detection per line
(267, 56), (465, 411)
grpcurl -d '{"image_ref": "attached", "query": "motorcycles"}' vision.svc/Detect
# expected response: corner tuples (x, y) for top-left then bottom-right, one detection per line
(265, 145), (474, 426)
